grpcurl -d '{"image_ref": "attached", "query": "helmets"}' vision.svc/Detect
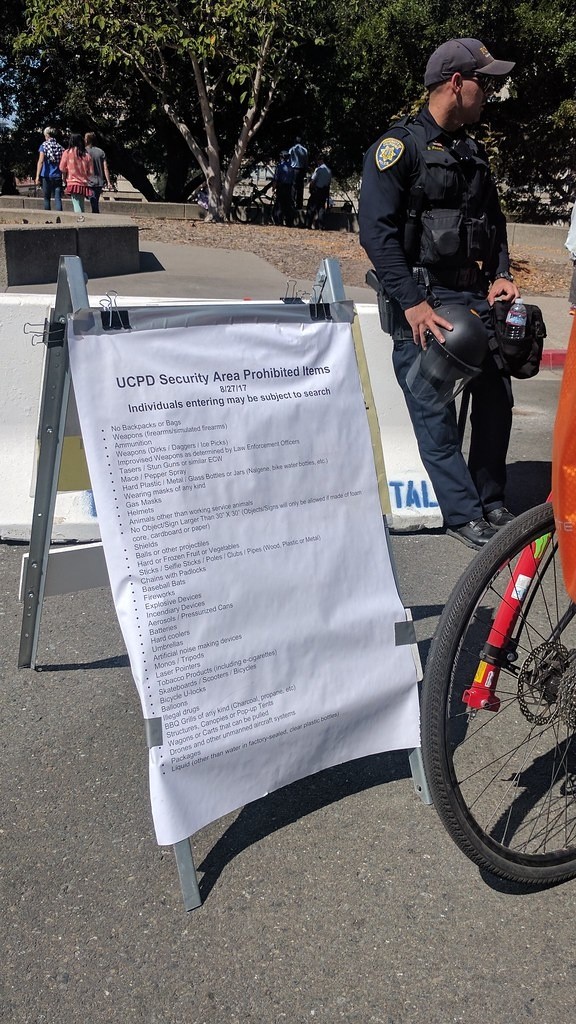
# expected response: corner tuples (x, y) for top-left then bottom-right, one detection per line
(419, 303), (489, 381)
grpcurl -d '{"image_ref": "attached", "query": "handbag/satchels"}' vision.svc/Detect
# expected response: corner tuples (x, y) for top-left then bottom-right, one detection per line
(494, 300), (547, 380)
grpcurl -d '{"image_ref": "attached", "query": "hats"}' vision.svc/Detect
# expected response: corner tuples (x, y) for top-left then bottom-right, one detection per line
(424, 37), (516, 87)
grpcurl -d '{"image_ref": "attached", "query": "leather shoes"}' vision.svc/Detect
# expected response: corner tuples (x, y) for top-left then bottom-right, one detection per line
(483, 507), (517, 529)
(446, 519), (499, 552)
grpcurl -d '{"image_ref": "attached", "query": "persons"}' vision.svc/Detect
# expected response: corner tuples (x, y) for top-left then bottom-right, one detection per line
(288, 137), (308, 209)
(304, 154), (331, 230)
(36, 126), (67, 212)
(358, 38), (514, 552)
(84, 132), (112, 213)
(273, 151), (295, 227)
(564, 199), (576, 315)
(59, 134), (94, 212)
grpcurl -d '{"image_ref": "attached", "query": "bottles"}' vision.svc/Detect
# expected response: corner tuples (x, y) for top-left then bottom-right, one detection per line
(504, 298), (527, 339)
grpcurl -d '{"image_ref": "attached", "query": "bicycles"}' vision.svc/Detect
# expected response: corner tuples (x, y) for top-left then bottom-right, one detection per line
(419, 499), (576, 889)
(233, 176), (279, 224)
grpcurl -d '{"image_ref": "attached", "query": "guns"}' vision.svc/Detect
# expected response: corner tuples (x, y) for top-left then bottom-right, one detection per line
(363, 269), (393, 334)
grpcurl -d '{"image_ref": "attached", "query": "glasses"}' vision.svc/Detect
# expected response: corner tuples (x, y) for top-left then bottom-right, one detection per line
(442, 70), (494, 93)
